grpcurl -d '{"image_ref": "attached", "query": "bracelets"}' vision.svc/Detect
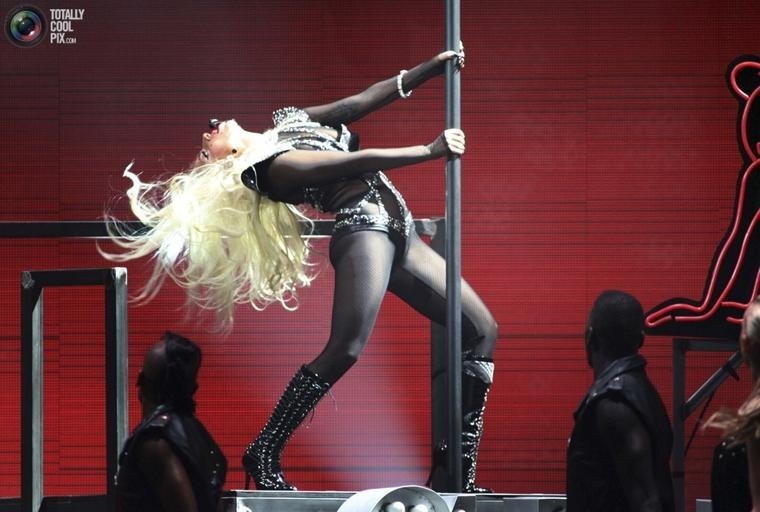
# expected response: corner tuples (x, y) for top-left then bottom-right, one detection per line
(395, 68), (412, 99)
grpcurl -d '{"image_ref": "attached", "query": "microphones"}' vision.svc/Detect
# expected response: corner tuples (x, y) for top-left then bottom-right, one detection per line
(208, 119), (221, 128)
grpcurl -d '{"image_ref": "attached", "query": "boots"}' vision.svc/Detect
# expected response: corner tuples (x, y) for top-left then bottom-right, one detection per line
(242, 364), (332, 490)
(425, 355), (493, 492)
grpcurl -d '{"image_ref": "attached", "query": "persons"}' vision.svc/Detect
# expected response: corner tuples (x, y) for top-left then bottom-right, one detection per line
(97, 39), (499, 494)
(563, 291), (672, 512)
(114, 331), (228, 511)
(700, 300), (760, 511)
